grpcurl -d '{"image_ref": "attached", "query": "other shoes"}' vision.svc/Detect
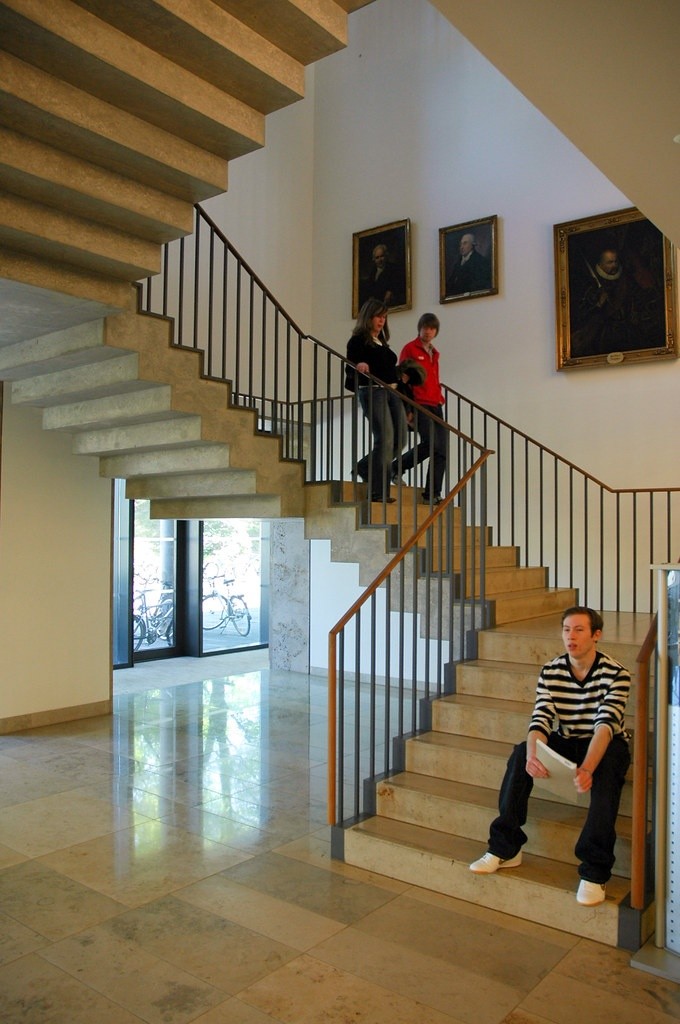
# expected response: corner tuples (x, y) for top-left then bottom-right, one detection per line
(355, 464), (368, 482)
(371, 496), (397, 504)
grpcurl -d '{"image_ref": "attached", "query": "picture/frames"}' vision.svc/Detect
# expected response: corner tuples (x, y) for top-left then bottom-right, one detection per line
(439, 215), (498, 305)
(351, 217), (412, 320)
(553, 206), (680, 372)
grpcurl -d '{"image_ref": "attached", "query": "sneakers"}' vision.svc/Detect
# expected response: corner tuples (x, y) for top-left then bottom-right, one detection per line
(421, 496), (443, 504)
(469, 849), (524, 873)
(577, 880), (605, 906)
(390, 471), (407, 486)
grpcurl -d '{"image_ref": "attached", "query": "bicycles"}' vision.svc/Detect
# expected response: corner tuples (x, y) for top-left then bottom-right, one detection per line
(133, 573), (173, 651)
(203, 575), (252, 637)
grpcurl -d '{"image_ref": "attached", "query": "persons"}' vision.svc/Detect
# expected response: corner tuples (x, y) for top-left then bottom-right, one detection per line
(398, 314), (448, 505)
(468, 606), (632, 906)
(344, 298), (409, 503)
